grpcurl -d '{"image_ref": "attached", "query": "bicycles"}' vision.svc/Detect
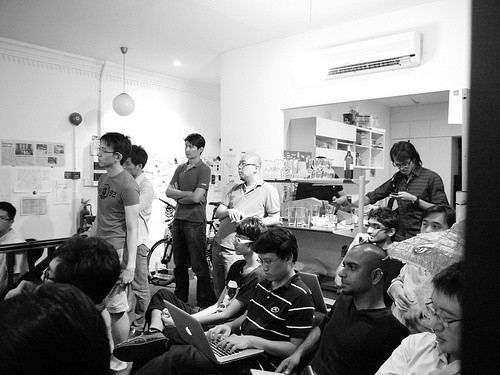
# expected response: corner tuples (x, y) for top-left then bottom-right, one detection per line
(146, 198), (223, 287)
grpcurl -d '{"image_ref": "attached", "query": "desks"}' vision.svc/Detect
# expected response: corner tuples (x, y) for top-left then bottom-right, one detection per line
(276, 223), (370, 238)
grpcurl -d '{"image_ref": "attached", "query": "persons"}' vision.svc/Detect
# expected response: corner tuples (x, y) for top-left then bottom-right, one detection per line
(122, 144), (154, 340)
(332, 141), (454, 241)
(78, 133), (140, 374)
(295, 156), (347, 208)
(213, 152), (280, 299)
(166, 133), (212, 310)
(0, 201), (467, 375)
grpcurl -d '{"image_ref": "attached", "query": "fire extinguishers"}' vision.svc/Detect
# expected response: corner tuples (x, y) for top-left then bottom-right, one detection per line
(79, 198), (91, 238)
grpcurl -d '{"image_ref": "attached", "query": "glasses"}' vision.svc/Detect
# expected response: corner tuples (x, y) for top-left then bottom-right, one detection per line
(364, 221), (388, 231)
(41, 267), (57, 284)
(255, 256), (281, 265)
(424, 301), (463, 328)
(392, 159), (412, 167)
(234, 235), (254, 244)
(1, 217), (10, 222)
(96, 146), (116, 154)
(237, 162), (257, 168)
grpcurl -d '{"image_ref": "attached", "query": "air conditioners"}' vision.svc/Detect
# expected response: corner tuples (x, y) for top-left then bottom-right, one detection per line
(320, 32), (423, 82)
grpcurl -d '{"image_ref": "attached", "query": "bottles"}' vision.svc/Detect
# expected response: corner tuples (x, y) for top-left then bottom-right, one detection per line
(356, 153), (362, 166)
(343, 146), (354, 179)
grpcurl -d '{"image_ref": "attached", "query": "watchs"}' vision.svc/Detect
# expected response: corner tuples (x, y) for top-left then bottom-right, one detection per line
(414, 197), (419, 205)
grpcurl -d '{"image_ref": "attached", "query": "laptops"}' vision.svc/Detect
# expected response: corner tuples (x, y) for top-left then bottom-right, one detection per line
(163, 299), (264, 365)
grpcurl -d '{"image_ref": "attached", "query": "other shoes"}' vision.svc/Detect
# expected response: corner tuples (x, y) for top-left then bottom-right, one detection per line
(113, 327), (170, 362)
(127, 328), (144, 339)
(109, 356), (128, 371)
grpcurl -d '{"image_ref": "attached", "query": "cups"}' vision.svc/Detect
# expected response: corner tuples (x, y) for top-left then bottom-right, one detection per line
(343, 113), (353, 124)
(287, 205), (335, 229)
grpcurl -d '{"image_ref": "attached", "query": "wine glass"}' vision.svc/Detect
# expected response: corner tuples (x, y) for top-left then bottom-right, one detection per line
(261, 159), (336, 179)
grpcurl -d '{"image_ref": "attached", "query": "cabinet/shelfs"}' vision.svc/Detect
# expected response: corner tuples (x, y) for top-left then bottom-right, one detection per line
(290, 116), (386, 203)
